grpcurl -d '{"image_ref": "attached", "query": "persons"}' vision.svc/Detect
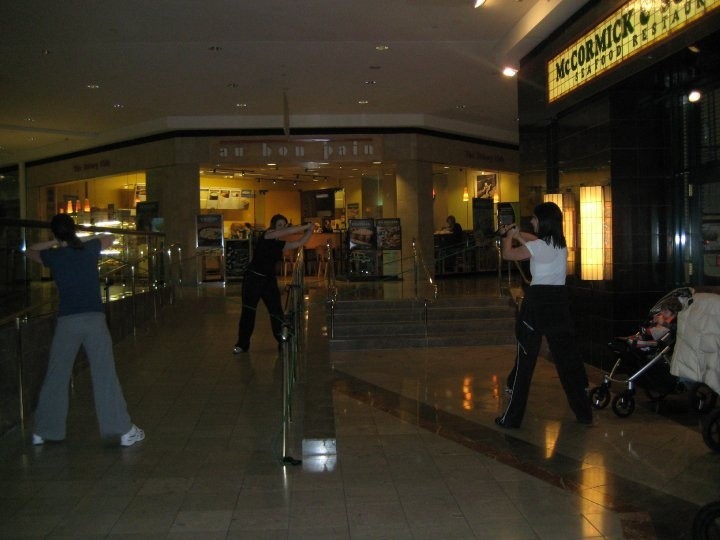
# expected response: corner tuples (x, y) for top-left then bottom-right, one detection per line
(23, 213), (147, 447)
(442, 214), (464, 270)
(503, 248), (593, 395)
(232, 213), (318, 354)
(623, 297), (683, 354)
(493, 202), (596, 428)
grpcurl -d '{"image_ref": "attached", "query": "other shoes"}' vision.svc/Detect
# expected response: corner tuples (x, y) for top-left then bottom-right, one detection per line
(233, 347), (243, 354)
(574, 414), (593, 425)
(583, 386), (592, 393)
(494, 417), (520, 429)
(504, 386), (514, 394)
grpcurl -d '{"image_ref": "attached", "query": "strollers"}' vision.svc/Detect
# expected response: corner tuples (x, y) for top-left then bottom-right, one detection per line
(589, 288), (695, 418)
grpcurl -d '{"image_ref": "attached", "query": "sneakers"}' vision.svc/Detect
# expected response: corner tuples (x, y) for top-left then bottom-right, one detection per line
(121, 424), (145, 446)
(32, 433), (44, 445)
